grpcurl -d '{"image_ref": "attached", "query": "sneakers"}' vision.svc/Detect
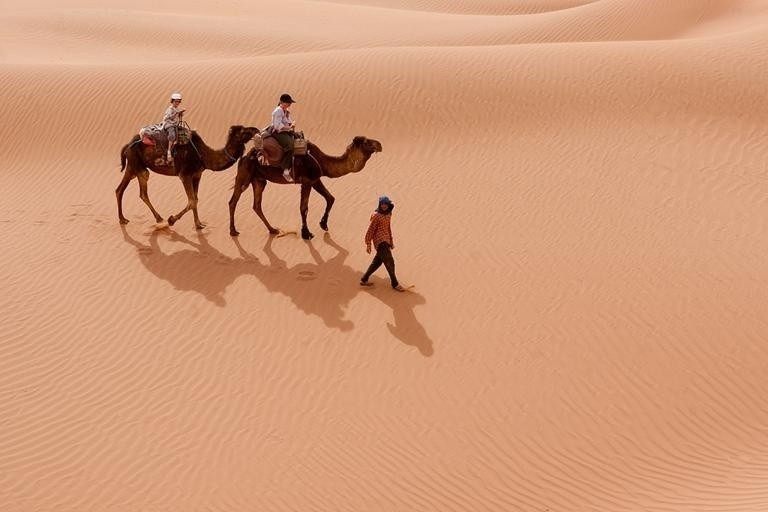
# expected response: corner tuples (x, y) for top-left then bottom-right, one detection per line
(282, 173), (294, 183)
(393, 283), (406, 292)
(167, 154), (174, 161)
(360, 281), (374, 287)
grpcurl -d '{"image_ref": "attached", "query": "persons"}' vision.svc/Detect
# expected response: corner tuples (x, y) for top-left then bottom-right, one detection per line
(162, 93), (185, 164)
(272, 93), (295, 183)
(358, 194), (406, 292)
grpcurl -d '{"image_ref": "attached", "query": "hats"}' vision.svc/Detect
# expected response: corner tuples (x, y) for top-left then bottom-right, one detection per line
(171, 93), (182, 100)
(280, 94), (295, 103)
(379, 196), (389, 204)
